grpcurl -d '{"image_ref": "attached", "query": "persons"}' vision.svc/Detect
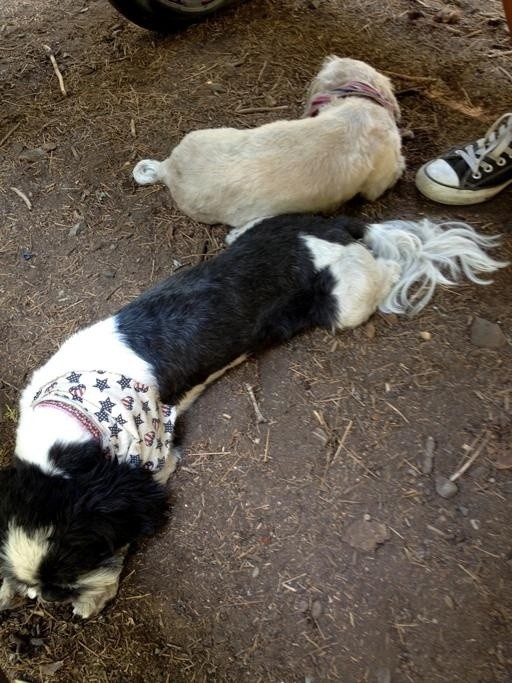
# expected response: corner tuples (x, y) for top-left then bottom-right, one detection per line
(414, 0), (512, 207)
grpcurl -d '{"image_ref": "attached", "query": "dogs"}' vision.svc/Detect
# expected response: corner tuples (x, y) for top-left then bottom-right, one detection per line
(0, 211), (512, 623)
(132, 52), (408, 227)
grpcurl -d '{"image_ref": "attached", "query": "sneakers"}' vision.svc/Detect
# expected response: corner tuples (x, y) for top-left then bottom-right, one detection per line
(414, 112), (512, 206)
